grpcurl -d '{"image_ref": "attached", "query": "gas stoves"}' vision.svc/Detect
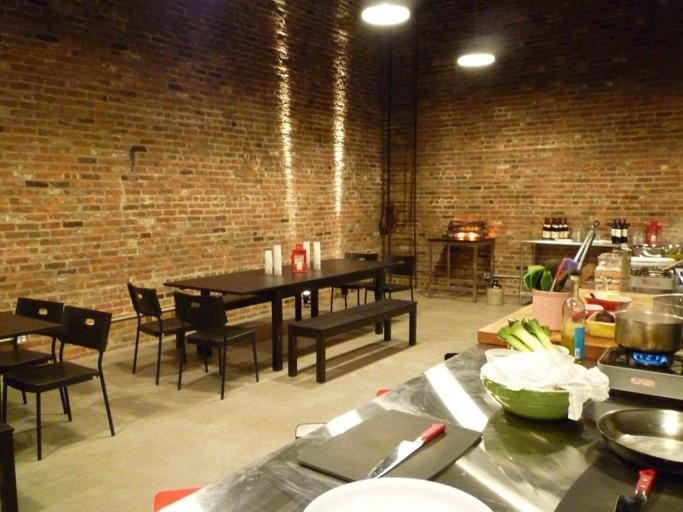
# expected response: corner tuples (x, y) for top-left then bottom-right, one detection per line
(596, 347), (683, 399)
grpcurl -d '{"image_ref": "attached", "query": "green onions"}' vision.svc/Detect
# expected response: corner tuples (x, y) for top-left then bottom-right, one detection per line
(496, 318), (559, 350)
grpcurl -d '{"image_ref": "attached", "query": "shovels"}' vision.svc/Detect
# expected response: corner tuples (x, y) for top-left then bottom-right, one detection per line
(615, 469), (655, 512)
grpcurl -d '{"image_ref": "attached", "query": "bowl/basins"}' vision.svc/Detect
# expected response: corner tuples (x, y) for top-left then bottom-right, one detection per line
(632, 242), (681, 259)
(584, 293), (632, 311)
(486, 288), (504, 305)
(531, 286), (569, 332)
(614, 292), (683, 352)
(480, 360), (596, 422)
(596, 408), (683, 474)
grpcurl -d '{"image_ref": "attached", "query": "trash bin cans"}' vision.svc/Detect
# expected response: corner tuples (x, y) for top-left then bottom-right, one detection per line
(487, 286), (503, 306)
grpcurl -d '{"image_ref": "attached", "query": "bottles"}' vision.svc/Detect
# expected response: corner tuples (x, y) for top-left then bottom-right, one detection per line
(290, 244), (308, 273)
(540, 214), (569, 241)
(592, 243), (631, 293)
(560, 269), (584, 362)
(610, 218), (628, 243)
(488, 279), (501, 289)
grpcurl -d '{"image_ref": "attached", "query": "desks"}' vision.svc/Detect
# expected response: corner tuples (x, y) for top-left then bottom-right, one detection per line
(427, 237), (496, 304)
(518, 239), (628, 306)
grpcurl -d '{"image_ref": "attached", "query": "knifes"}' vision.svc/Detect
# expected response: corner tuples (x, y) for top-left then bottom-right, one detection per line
(610, 465), (656, 510)
(366, 422), (445, 479)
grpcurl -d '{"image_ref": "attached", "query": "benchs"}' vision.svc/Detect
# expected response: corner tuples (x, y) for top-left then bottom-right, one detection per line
(285, 296), (416, 384)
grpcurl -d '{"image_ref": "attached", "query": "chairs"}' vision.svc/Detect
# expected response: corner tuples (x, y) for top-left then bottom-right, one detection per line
(170, 288), (261, 400)
(124, 281), (212, 384)
(0, 290), (118, 462)
(159, 251), (415, 372)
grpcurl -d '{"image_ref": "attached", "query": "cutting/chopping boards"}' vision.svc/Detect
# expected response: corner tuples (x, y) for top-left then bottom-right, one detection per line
(297, 409), (482, 481)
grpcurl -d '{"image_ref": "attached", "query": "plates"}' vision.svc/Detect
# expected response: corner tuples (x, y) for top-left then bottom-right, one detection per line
(302, 474), (491, 512)
